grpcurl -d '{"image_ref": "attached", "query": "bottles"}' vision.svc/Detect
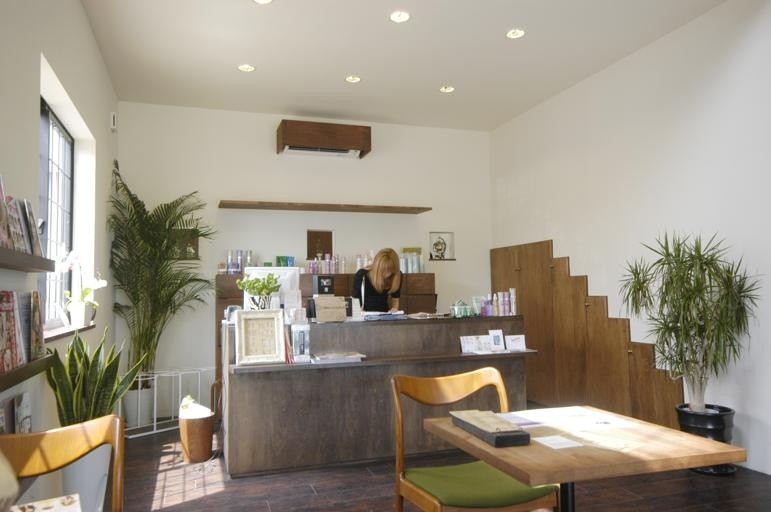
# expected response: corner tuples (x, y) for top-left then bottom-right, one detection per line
(498, 287), (517, 316)
(226, 247), (253, 274)
(400, 247), (425, 273)
(310, 252), (368, 274)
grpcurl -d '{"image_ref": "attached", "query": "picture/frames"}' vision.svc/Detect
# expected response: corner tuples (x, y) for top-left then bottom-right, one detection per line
(235, 310), (285, 365)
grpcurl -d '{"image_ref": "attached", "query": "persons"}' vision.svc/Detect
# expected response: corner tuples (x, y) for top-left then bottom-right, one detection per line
(352, 248), (402, 313)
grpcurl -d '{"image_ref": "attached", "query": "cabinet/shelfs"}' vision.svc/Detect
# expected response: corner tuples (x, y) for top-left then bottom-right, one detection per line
(1, 249), (54, 392)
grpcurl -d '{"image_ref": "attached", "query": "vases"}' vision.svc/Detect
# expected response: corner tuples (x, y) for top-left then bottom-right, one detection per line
(70, 302), (93, 326)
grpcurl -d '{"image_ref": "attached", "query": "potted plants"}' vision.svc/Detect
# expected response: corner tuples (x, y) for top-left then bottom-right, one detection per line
(618, 227), (764, 475)
(109, 158), (223, 428)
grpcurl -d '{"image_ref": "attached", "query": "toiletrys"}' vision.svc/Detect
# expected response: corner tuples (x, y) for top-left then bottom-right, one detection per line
(218, 250), (424, 274)
(450, 288), (516, 318)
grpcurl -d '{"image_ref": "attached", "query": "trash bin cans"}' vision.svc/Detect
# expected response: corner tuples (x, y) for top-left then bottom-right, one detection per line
(178, 411), (215, 463)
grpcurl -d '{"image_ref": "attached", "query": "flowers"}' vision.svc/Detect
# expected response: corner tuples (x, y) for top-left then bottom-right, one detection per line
(54, 243), (107, 329)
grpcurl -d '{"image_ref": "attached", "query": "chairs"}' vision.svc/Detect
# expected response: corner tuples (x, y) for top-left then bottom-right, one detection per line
(2, 412), (124, 510)
(390, 366), (560, 512)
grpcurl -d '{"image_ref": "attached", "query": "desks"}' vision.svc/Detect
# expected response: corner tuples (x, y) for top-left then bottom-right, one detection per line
(424, 405), (744, 512)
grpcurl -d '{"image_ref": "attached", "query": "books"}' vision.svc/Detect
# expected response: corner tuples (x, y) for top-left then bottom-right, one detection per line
(0, 196), (46, 433)
(310, 352), (367, 364)
